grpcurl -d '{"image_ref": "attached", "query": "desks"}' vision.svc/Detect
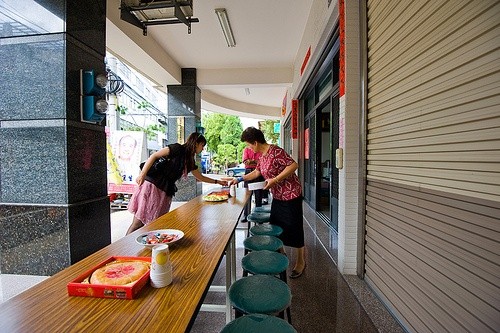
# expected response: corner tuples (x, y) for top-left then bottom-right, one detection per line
(0, 187), (253, 333)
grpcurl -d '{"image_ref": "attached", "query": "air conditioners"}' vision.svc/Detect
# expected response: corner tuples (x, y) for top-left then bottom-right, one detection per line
(124, 0), (193, 20)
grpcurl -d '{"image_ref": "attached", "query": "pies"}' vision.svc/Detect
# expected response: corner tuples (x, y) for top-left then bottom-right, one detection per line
(90, 261), (149, 285)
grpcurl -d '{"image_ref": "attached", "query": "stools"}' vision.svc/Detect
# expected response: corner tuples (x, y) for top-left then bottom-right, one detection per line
(243, 234), (284, 255)
(241, 249), (290, 285)
(246, 211), (271, 237)
(248, 224), (284, 236)
(219, 313), (299, 333)
(253, 206), (271, 213)
(227, 273), (292, 325)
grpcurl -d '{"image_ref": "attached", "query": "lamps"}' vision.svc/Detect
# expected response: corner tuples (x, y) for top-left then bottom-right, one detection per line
(78, 67), (109, 126)
(215, 7), (236, 47)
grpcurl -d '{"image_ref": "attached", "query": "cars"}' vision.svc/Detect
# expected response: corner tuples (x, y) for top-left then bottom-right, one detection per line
(224, 164), (246, 177)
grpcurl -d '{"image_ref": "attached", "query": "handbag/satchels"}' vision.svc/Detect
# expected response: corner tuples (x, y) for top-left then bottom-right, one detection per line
(140, 156), (176, 181)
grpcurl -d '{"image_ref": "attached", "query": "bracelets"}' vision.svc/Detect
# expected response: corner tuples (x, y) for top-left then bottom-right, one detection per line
(238, 177), (244, 182)
(215, 180), (217, 184)
(274, 177), (279, 184)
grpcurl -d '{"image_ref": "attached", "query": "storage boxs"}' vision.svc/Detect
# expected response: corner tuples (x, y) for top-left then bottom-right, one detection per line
(66, 254), (153, 301)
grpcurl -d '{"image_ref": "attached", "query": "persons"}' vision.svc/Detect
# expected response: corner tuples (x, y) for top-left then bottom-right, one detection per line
(230, 127), (306, 278)
(107, 135), (137, 175)
(126, 132), (228, 235)
(241, 146), (269, 223)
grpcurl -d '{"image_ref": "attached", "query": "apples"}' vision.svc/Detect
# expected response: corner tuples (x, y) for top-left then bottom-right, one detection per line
(156, 252), (168, 264)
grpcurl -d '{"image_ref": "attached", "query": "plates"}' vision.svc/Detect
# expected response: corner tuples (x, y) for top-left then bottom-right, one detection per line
(135, 229), (185, 250)
(208, 191), (230, 197)
(202, 195), (229, 202)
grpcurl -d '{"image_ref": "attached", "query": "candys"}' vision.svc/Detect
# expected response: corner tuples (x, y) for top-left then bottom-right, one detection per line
(141, 232), (177, 244)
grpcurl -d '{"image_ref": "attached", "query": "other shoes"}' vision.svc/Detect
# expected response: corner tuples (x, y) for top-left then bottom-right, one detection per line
(241, 217), (247, 222)
(284, 253), (287, 257)
(289, 261), (306, 278)
(262, 201), (268, 204)
(259, 222), (263, 224)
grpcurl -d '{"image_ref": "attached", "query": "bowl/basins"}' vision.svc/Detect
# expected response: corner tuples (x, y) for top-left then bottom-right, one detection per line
(247, 181), (267, 190)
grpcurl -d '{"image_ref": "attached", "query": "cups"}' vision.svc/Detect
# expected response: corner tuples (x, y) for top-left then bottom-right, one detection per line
(150, 243), (173, 288)
(229, 185), (237, 197)
